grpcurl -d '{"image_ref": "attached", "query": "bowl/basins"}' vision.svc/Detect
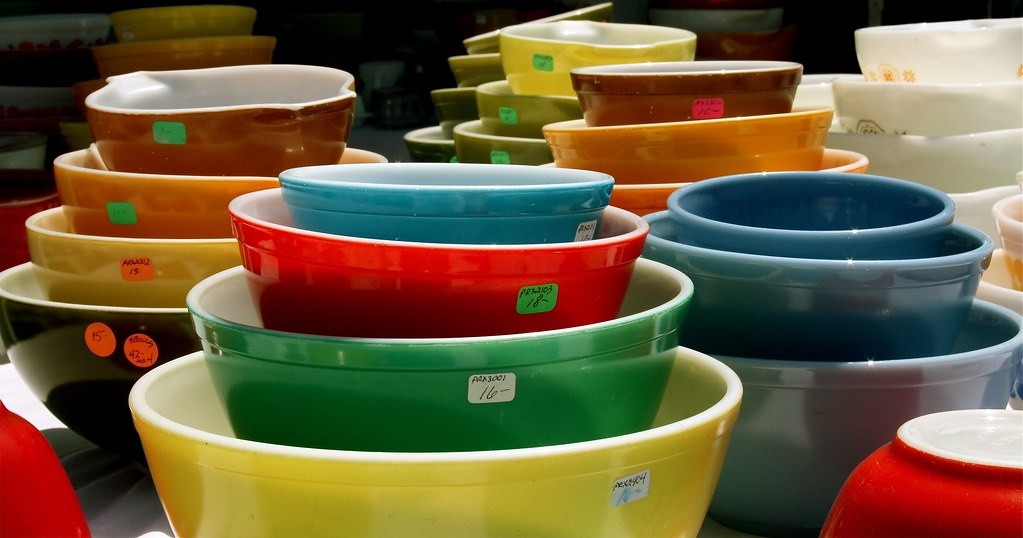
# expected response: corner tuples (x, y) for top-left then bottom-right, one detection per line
(1, 6), (1021, 538)
